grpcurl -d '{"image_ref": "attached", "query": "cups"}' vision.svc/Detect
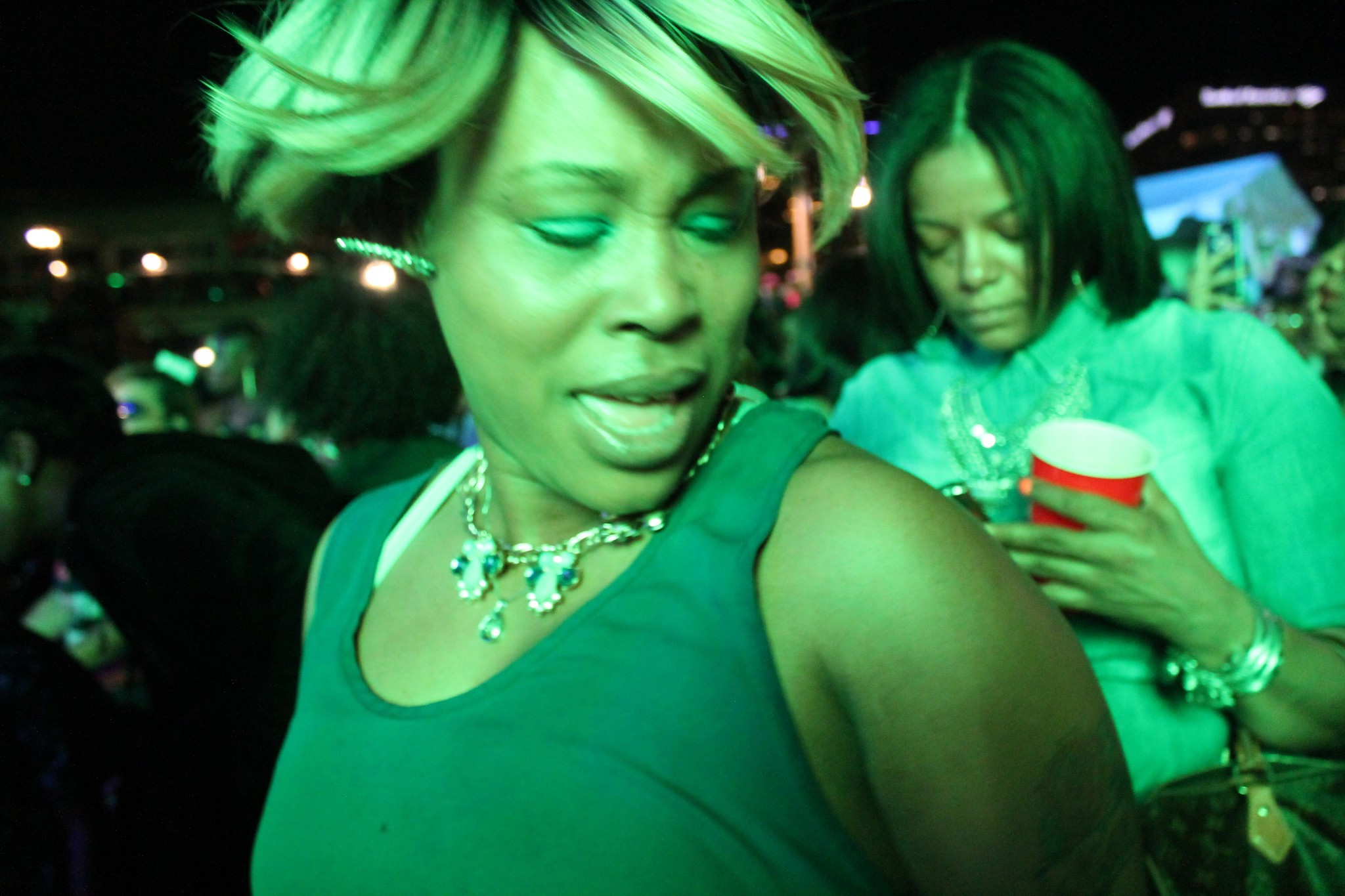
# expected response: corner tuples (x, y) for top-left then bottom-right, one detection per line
(1029, 418), (1159, 584)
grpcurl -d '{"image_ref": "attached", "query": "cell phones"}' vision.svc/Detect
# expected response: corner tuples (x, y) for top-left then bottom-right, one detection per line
(941, 483), (985, 525)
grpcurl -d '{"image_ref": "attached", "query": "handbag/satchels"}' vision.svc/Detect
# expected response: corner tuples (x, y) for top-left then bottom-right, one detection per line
(1135, 622), (1345, 896)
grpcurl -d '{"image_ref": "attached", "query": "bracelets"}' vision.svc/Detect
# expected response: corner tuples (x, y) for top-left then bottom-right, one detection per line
(1159, 599), (1281, 695)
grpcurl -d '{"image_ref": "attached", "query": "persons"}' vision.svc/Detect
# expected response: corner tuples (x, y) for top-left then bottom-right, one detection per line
(109, 360), (199, 436)
(0, 353), (345, 896)
(826, 43), (1345, 797)
(196, 0), (1145, 896)
(197, 319), (268, 437)
(0, 377), (190, 896)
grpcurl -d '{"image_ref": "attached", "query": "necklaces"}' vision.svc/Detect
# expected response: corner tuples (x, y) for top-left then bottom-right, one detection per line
(451, 381), (735, 641)
